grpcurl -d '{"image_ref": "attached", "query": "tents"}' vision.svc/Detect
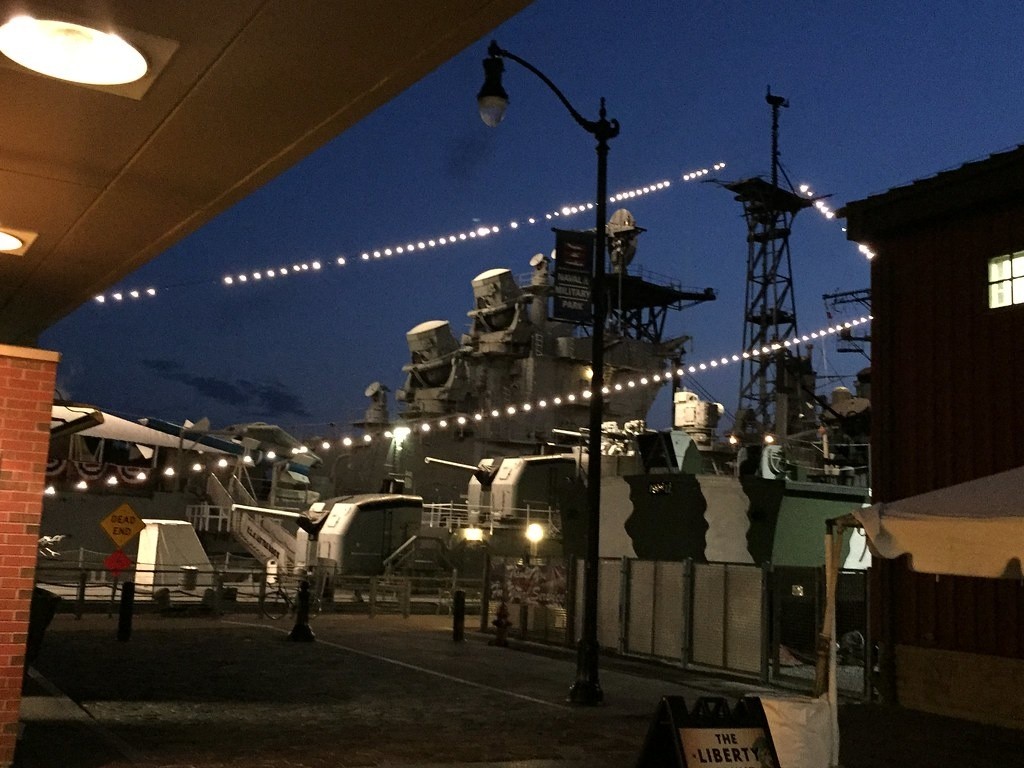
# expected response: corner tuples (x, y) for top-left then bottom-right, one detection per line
(822, 463), (1024, 768)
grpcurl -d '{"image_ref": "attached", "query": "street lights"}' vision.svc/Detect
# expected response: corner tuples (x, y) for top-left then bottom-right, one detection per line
(476, 39), (622, 704)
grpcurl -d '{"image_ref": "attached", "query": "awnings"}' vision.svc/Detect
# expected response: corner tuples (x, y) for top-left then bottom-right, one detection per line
(50, 403), (236, 457)
